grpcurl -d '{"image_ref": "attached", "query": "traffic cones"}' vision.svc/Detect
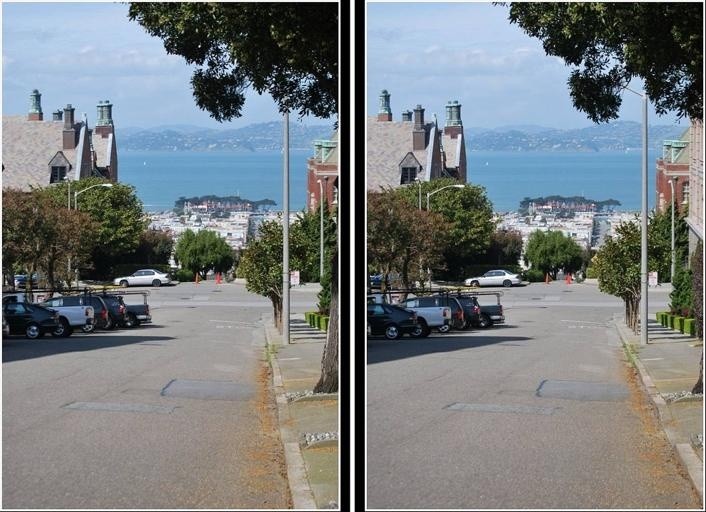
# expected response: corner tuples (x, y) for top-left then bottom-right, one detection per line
(194, 273), (200, 284)
(215, 272), (223, 284)
(566, 272), (571, 284)
(545, 272), (551, 284)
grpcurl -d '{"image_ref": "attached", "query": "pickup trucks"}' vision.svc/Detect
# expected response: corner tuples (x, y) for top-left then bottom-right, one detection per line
(2, 292), (97, 339)
(116, 304), (152, 329)
(395, 305), (453, 339)
(469, 303), (506, 329)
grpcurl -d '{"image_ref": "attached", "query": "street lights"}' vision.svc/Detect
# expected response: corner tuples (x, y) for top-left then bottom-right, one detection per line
(316, 178), (327, 285)
(413, 177), (467, 284)
(63, 176), (115, 281)
(668, 178), (677, 285)
(576, 71), (650, 346)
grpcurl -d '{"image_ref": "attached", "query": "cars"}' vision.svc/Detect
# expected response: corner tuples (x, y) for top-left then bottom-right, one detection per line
(9, 271), (48, 290)
(113, 268), (171, 287)
(368, 302), (418, 340)
(367, 272), (404, 290)
(2, 301), (59, 340)
(464, 269), (523, 287)
(88, 294), (129, 332)
(431, 294), (483, 332)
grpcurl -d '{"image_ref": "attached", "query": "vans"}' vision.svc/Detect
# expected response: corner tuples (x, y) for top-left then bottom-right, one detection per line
(34, 294), (110, 334)
(398, 295), (467, 334)
